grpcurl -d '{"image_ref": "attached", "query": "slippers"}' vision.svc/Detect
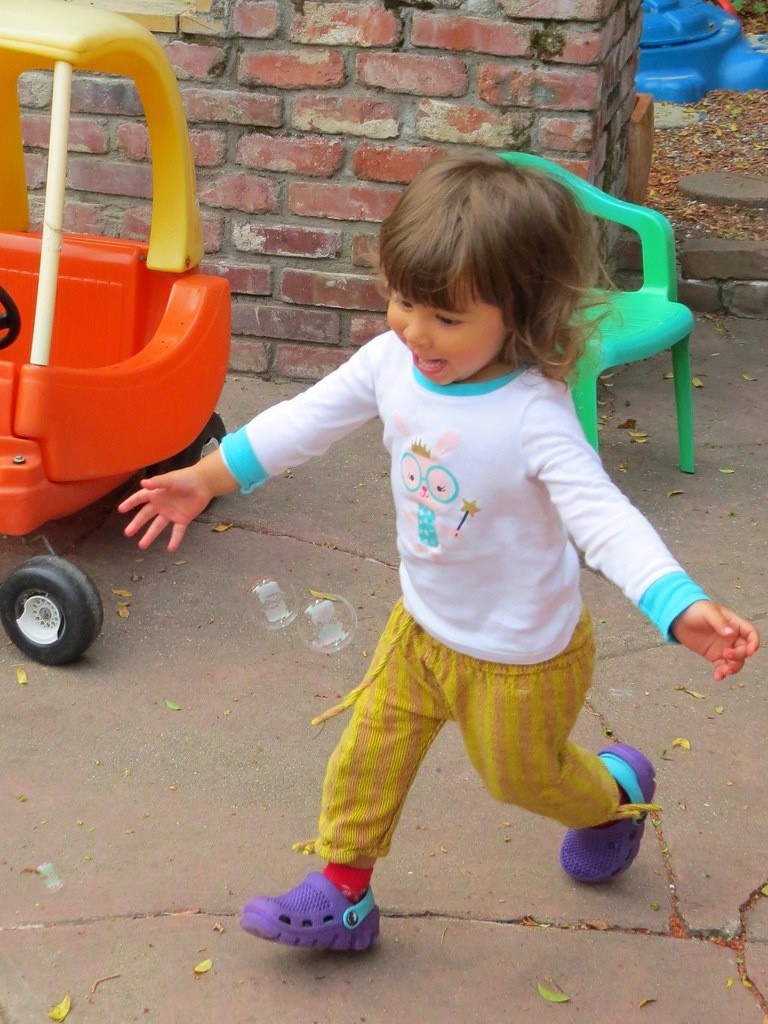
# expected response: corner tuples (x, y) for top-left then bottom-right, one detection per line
(239, 872), (380, 950)
(560, 743), (656, 885)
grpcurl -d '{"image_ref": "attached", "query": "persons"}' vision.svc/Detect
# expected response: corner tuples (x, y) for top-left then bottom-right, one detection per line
(115, 153), (759, 954)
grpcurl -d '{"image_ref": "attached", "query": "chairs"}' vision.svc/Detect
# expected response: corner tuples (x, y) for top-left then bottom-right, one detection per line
(496, 152), (699, 478)
